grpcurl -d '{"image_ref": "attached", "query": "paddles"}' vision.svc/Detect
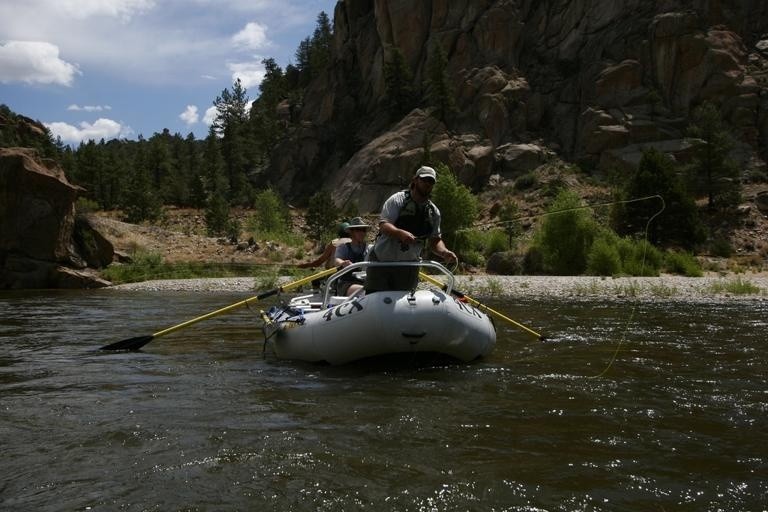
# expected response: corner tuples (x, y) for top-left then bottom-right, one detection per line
(100, 266), (344, 350)
(420, 271), (555, 341)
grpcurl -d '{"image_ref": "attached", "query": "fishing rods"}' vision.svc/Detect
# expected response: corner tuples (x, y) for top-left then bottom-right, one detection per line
(398, 195), (658, 244)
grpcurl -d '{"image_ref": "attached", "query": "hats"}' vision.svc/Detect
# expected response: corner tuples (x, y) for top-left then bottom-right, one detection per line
(344, 217), (370, 233)
(416, 166), (436, 182)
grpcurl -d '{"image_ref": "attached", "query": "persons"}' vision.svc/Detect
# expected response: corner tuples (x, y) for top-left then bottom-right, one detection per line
(331, 217), (375, 297)
(361, 165), (457, 296)
(295, 221), (352, 296)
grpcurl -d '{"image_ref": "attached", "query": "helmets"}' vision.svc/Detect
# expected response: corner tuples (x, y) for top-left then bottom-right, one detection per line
(337, 222), (349, 236)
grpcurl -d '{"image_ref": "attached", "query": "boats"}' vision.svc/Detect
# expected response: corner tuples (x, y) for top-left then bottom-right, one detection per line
(262, 260), (497, 368)
